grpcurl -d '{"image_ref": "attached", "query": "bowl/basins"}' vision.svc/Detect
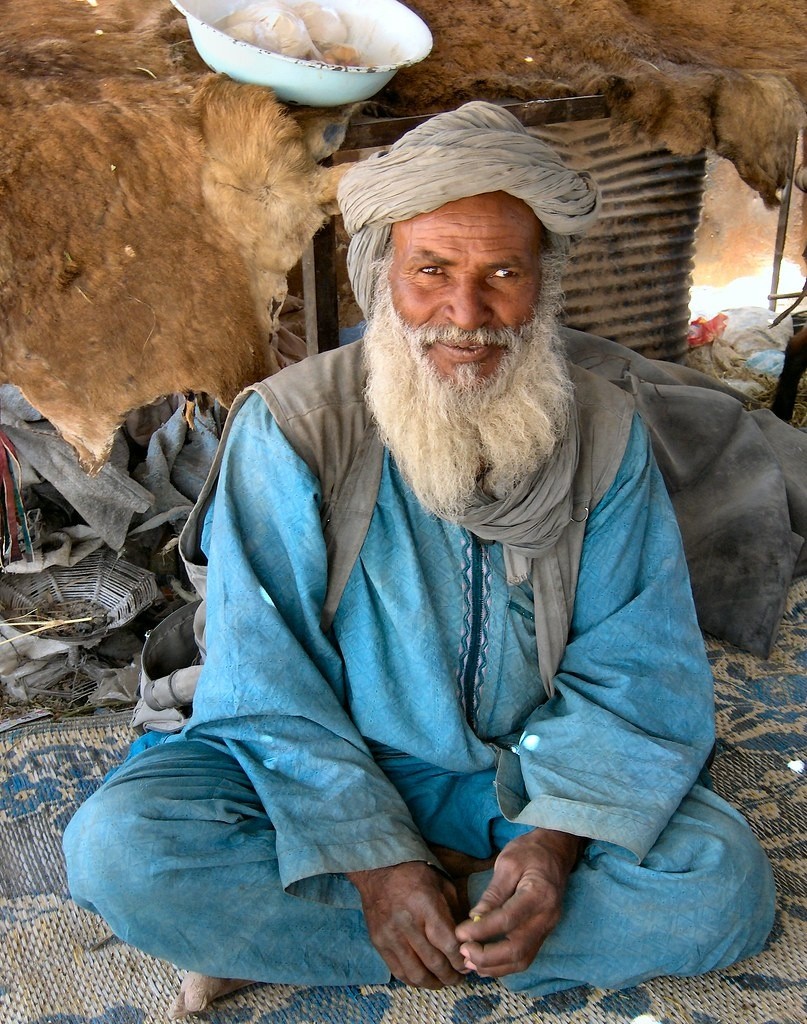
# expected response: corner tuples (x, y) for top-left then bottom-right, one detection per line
(166, 0), (433, 106)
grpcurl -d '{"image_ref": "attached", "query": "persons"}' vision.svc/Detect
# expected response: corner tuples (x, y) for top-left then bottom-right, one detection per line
(60, 101), (775, 1020)
